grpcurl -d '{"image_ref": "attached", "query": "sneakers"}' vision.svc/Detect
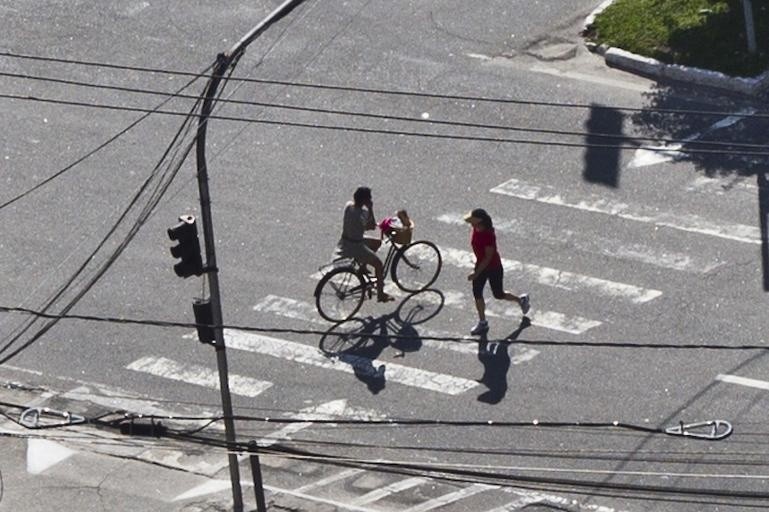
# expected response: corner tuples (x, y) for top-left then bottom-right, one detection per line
(376, 293), (397, 303)
(517, 292), (531, 316)
(468, 321), (490, 336)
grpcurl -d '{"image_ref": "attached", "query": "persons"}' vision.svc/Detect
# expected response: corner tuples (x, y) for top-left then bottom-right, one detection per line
(337, 187), (396, 304)
(463, 208), (533, 335)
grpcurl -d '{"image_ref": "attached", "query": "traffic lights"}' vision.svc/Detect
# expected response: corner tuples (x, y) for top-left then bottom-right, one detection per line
(167, 215), (202, 279)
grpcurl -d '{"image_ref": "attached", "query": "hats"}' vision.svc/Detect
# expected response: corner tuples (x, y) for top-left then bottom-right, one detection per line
(463, 207), (490, 226)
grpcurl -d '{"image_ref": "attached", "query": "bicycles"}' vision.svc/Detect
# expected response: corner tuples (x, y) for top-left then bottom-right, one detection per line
(311, 217), (443, 324)
